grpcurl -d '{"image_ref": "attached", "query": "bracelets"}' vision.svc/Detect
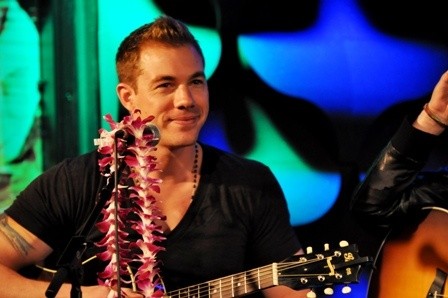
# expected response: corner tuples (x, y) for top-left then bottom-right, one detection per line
(424, 103), (447, 127)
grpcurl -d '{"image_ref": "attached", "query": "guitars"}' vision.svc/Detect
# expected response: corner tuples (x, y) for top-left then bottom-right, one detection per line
(367, 206), (448, 298)
(16, 239), (374, 298)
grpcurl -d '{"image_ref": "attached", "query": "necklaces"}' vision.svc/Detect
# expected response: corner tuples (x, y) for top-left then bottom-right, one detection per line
(192, 142), (198, 197)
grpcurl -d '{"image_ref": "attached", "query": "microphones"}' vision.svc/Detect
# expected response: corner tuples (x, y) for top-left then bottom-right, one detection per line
(115, 123), (161, 146)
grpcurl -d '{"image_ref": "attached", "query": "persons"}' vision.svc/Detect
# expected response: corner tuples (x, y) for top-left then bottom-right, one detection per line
(347, 70), (448, 239)
(0, 18), (313, 298)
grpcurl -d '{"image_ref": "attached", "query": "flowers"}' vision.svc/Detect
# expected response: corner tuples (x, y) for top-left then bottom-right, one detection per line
(93, 107), (168, 298)
